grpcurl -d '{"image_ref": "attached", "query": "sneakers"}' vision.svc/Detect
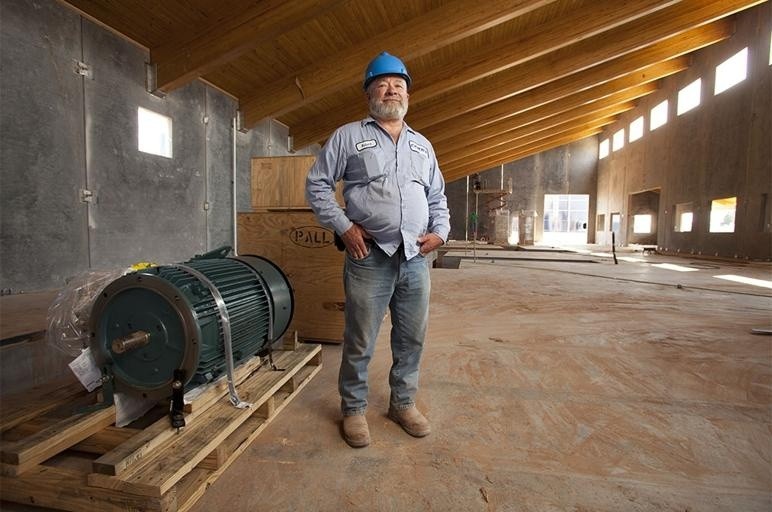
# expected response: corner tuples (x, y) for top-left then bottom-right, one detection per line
(389, 403), (431, 437)
(343, 413), (370, 447)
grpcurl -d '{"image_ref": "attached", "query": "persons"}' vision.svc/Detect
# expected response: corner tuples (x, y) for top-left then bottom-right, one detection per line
(304, 52), (452, 448)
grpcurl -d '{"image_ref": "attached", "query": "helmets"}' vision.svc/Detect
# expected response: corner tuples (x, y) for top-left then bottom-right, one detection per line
(363, 51), (412, 87)
